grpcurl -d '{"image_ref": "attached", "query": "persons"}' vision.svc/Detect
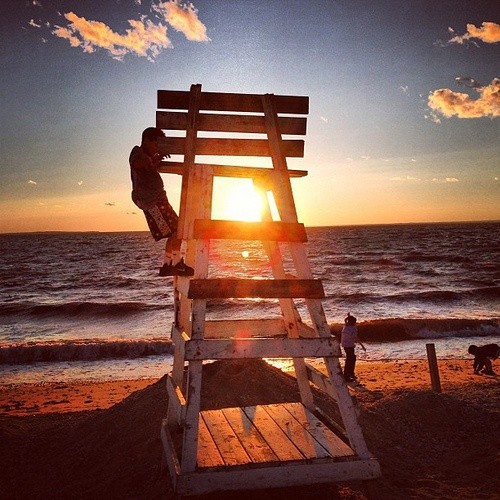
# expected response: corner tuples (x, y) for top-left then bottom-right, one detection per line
(128, 127), (194, 276)
(467, 343), (500, 375)
(340, 316), (366, 382)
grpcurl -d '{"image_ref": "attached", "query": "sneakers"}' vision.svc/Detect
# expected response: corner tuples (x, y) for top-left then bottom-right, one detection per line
(168, 257), (194, 276)
(157, 259), (172, 276)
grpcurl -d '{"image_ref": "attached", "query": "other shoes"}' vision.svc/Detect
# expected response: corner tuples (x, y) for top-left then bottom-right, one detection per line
(481, 369), (489, 373)
(345, 377), (356, 383)
(485, 371), (495, 375)
(351, 376), (360, 382)
